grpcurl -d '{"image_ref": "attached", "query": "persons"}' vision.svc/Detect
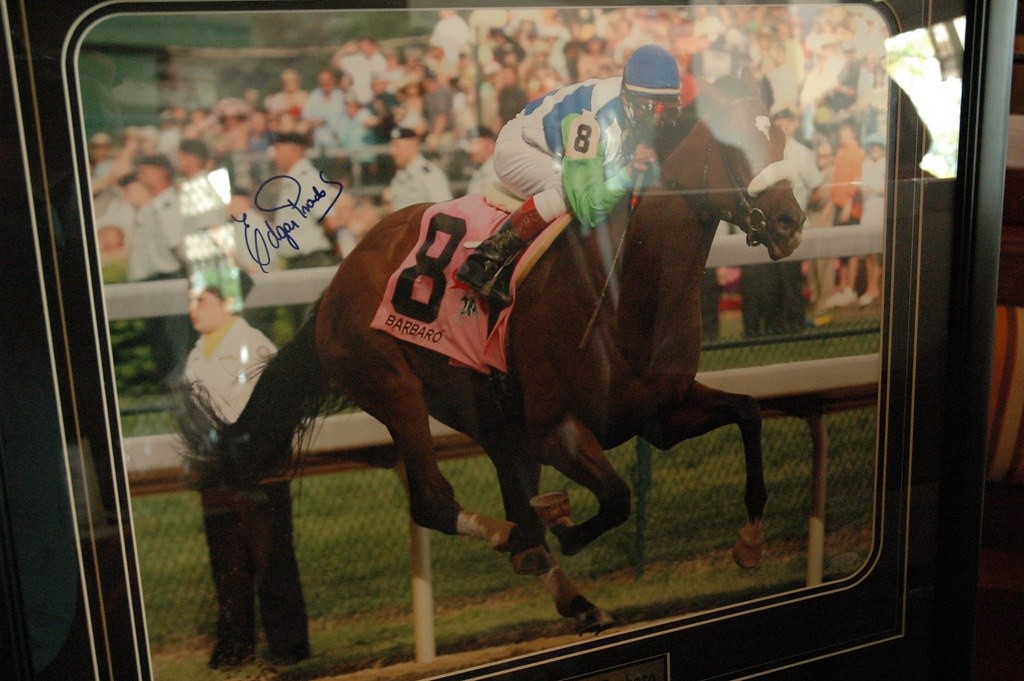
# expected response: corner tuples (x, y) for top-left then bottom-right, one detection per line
(83, 2), (891, 668)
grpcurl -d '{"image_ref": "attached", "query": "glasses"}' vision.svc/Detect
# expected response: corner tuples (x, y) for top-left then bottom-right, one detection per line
(632, 102), (675, 114)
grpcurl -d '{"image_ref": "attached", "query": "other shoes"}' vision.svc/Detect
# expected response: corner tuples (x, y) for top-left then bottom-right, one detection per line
(858, 292), (880, 307)
(825, 291), (858, 305)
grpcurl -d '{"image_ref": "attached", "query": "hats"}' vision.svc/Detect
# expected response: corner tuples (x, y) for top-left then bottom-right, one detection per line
(468, 127), (491, 138)
(267, 132), (307, 145)
(623, 43), (681, 96)
(89, 132), (110, 145)
(215, 97), (252, 118)
(388, 128), (415, 139)
(187, 262), (253, 304)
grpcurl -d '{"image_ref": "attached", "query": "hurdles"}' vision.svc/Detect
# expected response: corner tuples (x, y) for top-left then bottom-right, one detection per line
(121, 353), (883, 663)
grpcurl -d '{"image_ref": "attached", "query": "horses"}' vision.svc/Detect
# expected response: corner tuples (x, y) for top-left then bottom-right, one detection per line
(176, 66), (806, 638)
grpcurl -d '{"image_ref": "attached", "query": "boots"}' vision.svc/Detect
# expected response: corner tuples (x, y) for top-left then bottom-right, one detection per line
(456, 217), (526, 305)
